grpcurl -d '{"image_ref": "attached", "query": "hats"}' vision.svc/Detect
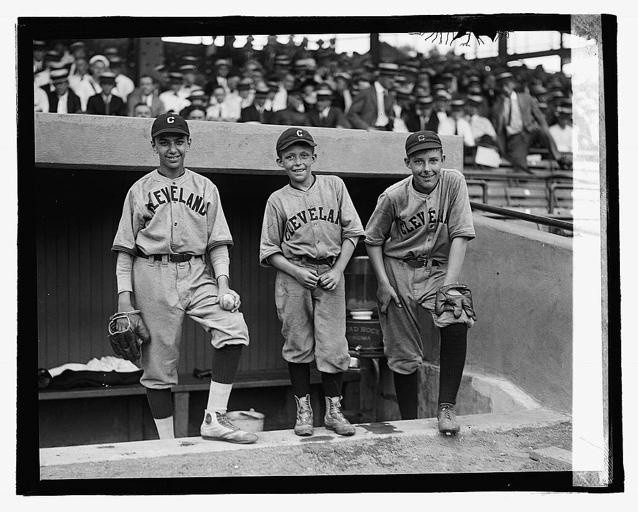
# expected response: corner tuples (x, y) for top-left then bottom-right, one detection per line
(467, 72), (571, 115)
(377, 61), (465, 109)
(276, 129), (315, 149)
(33, 40), (122, 87)
(152, 114), (189, 140)
(169, 56), (261, 102)
(276, 54), (352, 99)
(404, 131), (443, 156)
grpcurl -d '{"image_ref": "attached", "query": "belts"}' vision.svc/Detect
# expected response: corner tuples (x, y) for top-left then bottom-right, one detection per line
(301, 256), (335, 265)
(400, 256), (437, 269)
(137, 253), (192, 264)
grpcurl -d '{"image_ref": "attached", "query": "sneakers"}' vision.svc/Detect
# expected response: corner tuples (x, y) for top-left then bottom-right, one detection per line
(295, 395), (314, 435)
(437, 402), (460, 436)
(323, 396), (354, 435)
(198, 410), (258, 443)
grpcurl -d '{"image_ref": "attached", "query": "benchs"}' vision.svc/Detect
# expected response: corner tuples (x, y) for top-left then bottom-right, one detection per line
(38, 365), (363, 439)
(462, 154), (572, 238)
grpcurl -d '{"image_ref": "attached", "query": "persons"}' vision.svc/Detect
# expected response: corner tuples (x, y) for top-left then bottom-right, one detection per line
(110, 113), (259, 444)
(34, 34), (574, 182)
(365, 130), (477, 435)
(260, 127), (365, 436)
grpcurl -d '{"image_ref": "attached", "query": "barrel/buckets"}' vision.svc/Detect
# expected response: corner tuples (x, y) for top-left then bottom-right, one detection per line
(346, 257), (378, 311)
